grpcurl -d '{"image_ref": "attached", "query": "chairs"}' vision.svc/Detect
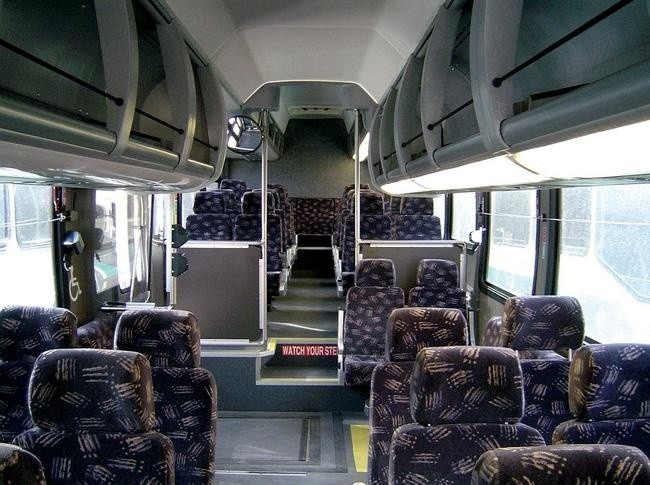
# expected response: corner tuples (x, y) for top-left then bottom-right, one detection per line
(1, 301), (220, 485)
(342, 255), (650, 484)
(184, 180), (444, 311)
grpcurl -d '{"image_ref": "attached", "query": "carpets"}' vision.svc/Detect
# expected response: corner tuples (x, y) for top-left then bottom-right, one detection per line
(349, 423), (372, 472)
